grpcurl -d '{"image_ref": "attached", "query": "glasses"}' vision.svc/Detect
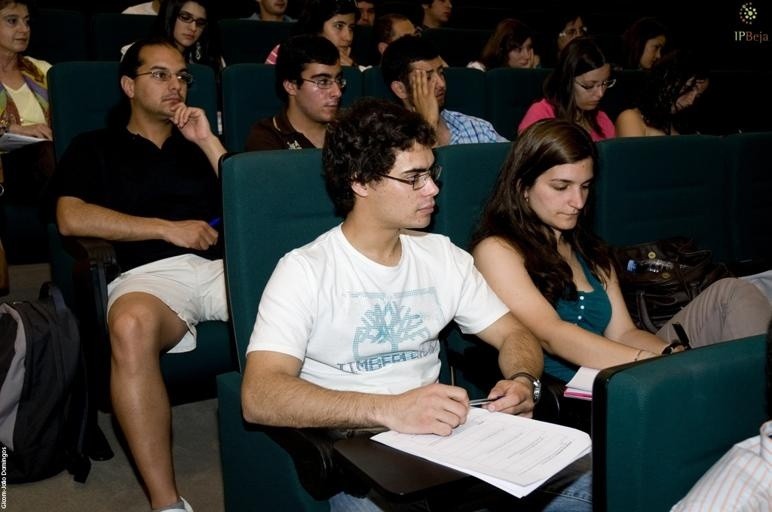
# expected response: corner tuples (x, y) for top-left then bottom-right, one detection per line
(559, 27), (587, 37)
(381, 166), (442, 190)
(575, 79), (616, 90)
(132, 71), (193, 84)
(177, 14), (209, 28)
(303, 78), (346, 89)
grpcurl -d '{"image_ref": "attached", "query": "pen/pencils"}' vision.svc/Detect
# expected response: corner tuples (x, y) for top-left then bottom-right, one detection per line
(208, 218), (220, 227)
(468, 396), (504, 406)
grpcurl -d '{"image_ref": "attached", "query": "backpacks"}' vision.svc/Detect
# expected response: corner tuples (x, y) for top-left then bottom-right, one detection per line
(0, 282), (114, 484)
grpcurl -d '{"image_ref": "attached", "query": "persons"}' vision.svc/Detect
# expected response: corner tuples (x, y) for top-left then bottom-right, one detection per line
(1, 3), (771, 512)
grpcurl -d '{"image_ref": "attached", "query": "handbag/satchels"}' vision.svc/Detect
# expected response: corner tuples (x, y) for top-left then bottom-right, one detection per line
(608, 235), (738, 334)
